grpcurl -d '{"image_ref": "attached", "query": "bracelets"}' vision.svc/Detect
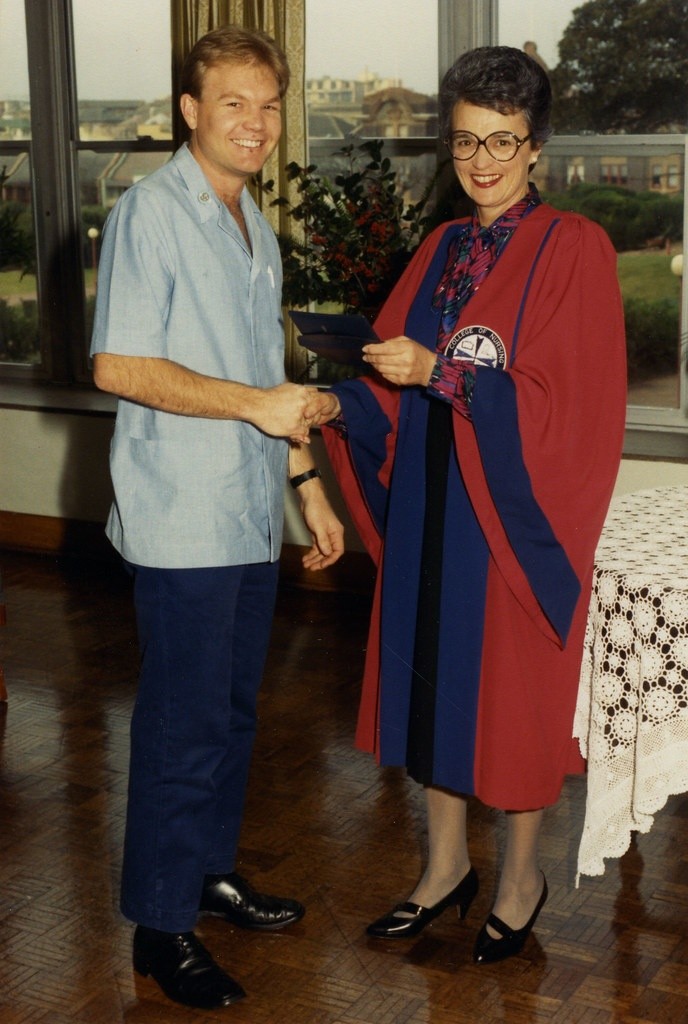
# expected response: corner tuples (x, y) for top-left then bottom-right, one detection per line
(288, 467), (322, 491)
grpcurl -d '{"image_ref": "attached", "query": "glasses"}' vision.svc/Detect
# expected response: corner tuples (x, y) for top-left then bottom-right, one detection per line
(444, 128), (534, 162)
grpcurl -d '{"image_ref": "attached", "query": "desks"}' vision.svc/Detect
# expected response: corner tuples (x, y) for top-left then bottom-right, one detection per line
(578, 462), (688, 923)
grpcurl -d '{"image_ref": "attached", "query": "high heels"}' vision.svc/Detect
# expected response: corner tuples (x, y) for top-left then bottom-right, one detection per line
(471, 870), (548, 965)
(365, 865), (479, 942)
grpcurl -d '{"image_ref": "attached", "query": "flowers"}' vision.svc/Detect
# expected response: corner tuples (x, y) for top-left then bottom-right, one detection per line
(279, 137), (429, 324)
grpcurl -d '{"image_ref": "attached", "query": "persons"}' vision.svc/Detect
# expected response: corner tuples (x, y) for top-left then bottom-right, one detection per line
(292, 45), (627, 964)
(86, 20), (344, 1011)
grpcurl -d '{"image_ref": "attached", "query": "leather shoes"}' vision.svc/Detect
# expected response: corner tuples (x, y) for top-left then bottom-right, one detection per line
(203, 873), (307, 931)
(131, 927), (245, 1011)
